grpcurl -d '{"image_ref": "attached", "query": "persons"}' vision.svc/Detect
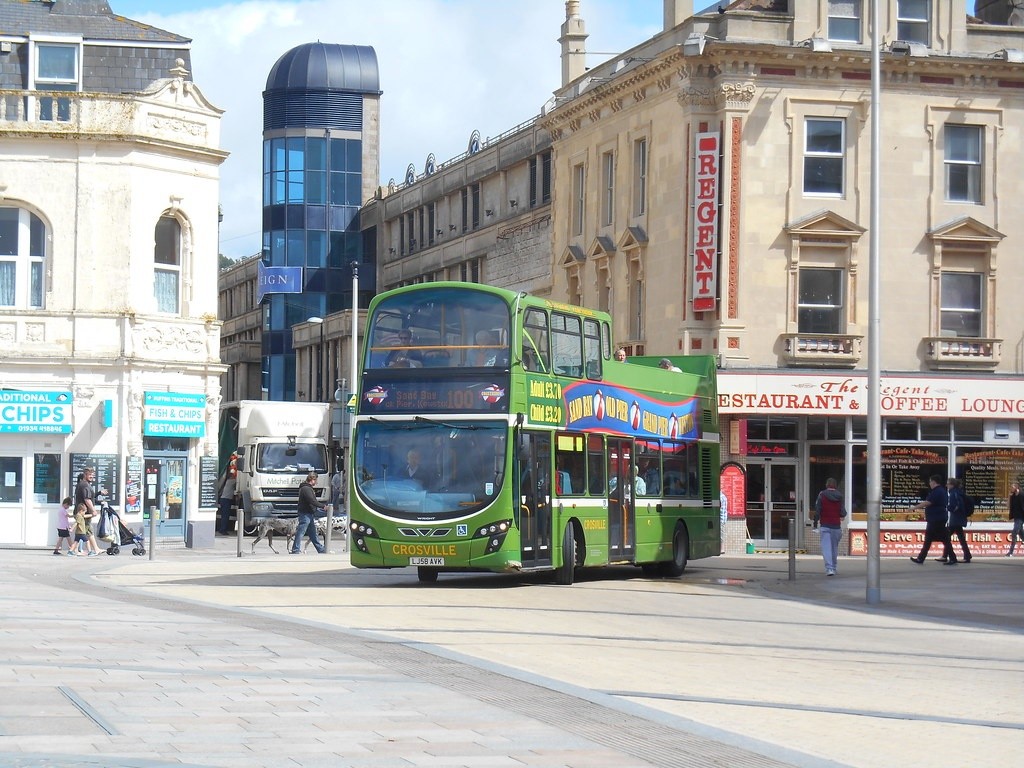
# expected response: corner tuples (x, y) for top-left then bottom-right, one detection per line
(53, 467), (109, 556)
(812, 478), (847, 575)
(658, 358), (683, 373)
(465, 331), (504, 366)
(935, 478), (972, 563)
(395, 448), (428, 490)
(218, 473), (238, 535)
(908, 475), (957, 566)
(290, 472), (327, 554)
(614, 347), (628, 364)
(386, 329), (423, 368)
(1004, 483), (1024, 556)
(609, 455), (659, 504)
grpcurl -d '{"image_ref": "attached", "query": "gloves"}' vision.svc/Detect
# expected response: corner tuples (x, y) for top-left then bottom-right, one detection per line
(813, 519), (817, 527)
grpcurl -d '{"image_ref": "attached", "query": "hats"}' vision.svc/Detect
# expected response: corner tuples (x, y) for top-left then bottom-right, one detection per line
(627, 463), (638, 474)
(639, 458), (649, 465)
(825, 478), (836, 489)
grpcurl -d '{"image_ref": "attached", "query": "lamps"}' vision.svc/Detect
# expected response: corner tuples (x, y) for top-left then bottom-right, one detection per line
(796, 38), (833, 53)
(574, 76), (613, 99)
(879, 41), (929, 58)
(541, 96), (576, 118)
(435, 230), (443, 236)
(485, 209), (494, 217)
(683, 33), (719, 56)
(609, 56), (654, 76)
(388, 248), (396, 255)
(298, 391), (304, 397)
(510, 200), (518, 207)
(411, 238), (416, 245)
(448, 225), (455, 231)
(986, 48), (1024, 63)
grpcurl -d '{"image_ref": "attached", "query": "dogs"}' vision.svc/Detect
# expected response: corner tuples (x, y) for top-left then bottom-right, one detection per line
(244, 517), (299, 554)
(303, 516), (348, 554)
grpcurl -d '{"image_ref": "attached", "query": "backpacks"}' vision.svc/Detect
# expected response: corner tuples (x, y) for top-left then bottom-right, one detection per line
(957, 490), (974, 517)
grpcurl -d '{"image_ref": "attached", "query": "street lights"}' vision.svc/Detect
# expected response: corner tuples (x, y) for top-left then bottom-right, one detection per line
(308, 317), (323, 401)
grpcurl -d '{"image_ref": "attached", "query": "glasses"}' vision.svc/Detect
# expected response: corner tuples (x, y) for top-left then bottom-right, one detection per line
(399, 336), (408, 339)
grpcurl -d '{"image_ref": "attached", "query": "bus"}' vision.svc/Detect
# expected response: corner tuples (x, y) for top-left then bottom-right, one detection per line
(345, 281), (723, 583)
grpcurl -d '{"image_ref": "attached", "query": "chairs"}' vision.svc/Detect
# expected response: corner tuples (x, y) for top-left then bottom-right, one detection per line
(426, 349), (450, 365)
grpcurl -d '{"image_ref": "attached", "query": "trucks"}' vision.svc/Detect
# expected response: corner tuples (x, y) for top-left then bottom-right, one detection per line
(218, 401), (344, 538)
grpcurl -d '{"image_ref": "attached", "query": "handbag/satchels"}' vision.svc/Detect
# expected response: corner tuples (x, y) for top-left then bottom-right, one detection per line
(96, 508), (121, 546)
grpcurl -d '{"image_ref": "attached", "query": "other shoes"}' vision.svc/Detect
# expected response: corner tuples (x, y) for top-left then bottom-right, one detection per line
(1005, 552), (1013, 557)
(910, 557), (923, 564)
(53, 550), (62, 555)
(88, 551), (97, 556)
(935, 556), (946, 562)
(96, 548), (107, 555)
(219, 528), (229, 535)
(827, 568), (837, 576)
(66, 550), (77, 557)
(945, 557), (957, 564)
(957, 557), (970, 563)
(74, 549), (84, 556)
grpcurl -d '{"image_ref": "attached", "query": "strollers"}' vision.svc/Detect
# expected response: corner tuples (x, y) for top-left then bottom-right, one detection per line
(96, 494), (146, 556)
